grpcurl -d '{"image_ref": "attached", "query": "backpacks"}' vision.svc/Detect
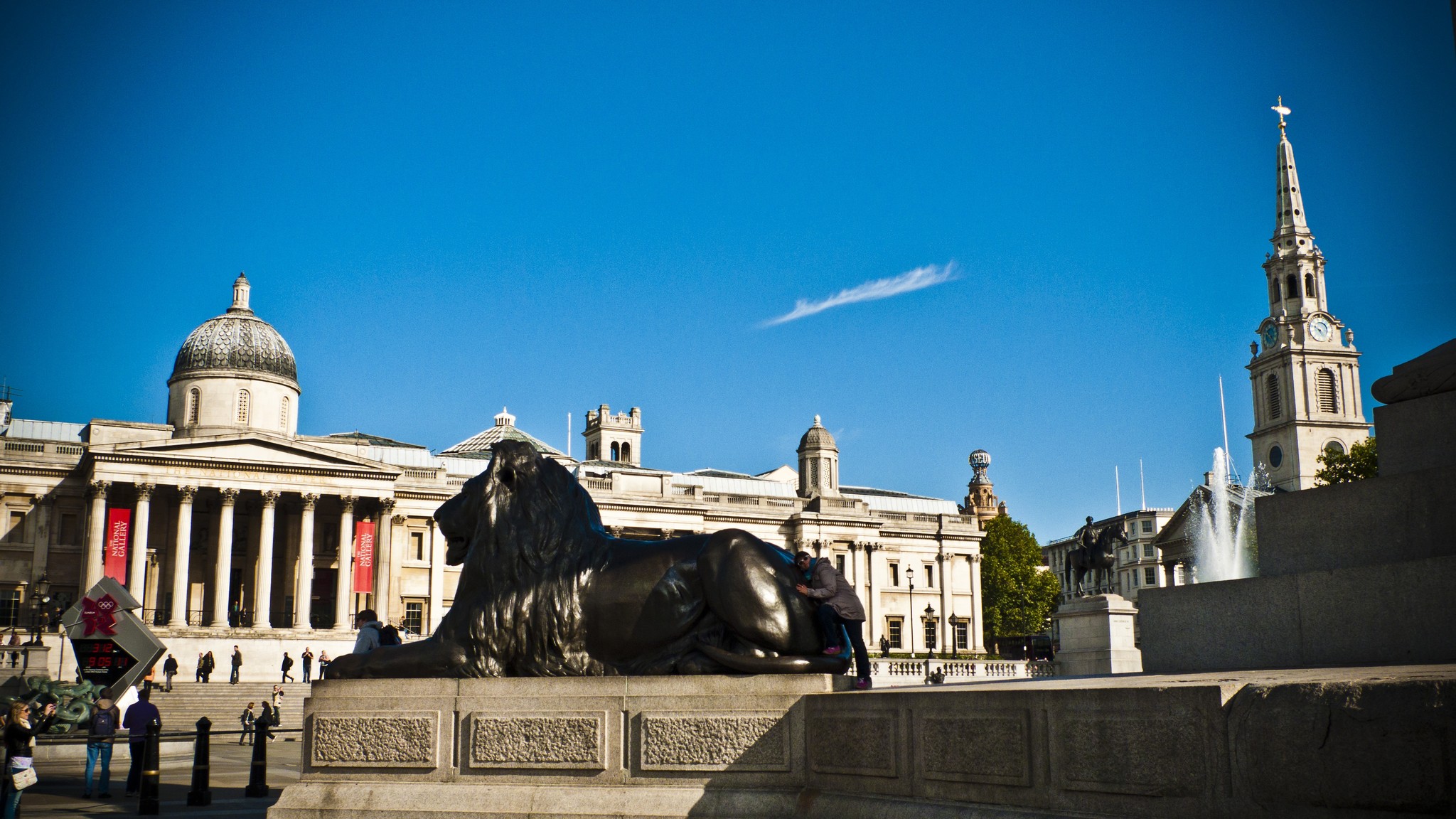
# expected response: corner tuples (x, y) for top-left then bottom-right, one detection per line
(288, 658), (294, 667)
(89, 703), (117, 738)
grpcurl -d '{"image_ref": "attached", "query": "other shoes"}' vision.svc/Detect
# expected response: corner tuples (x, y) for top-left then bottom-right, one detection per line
(308, 681), (311, 683)
(229, 681), (234, 684)
(166, 690), (170, 692)
(280, 682), (286, 684)
(170, 686), (173, 690)
(98, 792), (111, 799)
(82, 793), (97, 799)
(194, 681), (200, 683)
(125, 790), (140, 798)
(249, 742), (254, 746)
(236, 682), (239, 684)
(239, 742), (245, 745)
(202, 681), (208, 683)
(301, 681), (306, 683)
(291, 678), (294, 683)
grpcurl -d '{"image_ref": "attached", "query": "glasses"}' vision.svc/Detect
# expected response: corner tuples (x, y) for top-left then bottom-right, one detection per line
(21, 706), (33, 713)
(796, 555), (810, 565)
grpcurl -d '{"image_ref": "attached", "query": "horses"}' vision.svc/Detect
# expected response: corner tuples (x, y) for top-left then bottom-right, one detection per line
(1064, 523), (1129, 598)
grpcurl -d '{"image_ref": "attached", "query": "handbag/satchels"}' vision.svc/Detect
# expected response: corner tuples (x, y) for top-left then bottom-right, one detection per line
(267, 715), (274, 726)
(174, 670), (177, 675)
(324, 659), (330, 665)
(13, 767), (37, 790)
(238, 714), (244, 725)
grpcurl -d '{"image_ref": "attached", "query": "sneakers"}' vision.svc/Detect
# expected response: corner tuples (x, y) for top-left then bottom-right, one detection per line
(272, 736), (277, 743)
(278, 724), (282, 727)
(824, 644), (841, 655)
(855, 678), (868, 689)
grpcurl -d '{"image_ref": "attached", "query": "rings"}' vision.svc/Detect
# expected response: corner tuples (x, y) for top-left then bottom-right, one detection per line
(802, 586), (803, 588)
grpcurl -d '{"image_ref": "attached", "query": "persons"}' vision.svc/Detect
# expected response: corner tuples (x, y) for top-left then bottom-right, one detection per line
(281, 652), (294, 684)
(229, 644), (243, 684)
(1, 701), (57, 819)
(319, 650), (331, 680)
(261, 701), (277, 743)
(239, 702), (257, 746)
(79, 687), (121, 791)
(8, 631), (21, 668)
(163, 653), (178, 693)
(122, 688), (163, 795)
(0, 710), (6, 728)
(143, 666), (155, 699)
(879, 635), (888, 654)
(195, 653), (210, 683)
(795, 550), (874, 690)
(351, 609), (383, 655)
(393, 615), (412, 639)
(0, 634), (5, 665)
(278, 687), (284, 704)
(272, 685), (283, 727)
(1078, 516), (1102, 573)
(40, 612), (49, 633)
(202, 651), (217, 683)
(301, 647), (314, 684)
(230, 601), (240, 627)
(240, 606), (248, 627)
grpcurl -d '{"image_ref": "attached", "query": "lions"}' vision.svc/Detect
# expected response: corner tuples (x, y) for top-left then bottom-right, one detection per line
(325, 437), (854, 677)
